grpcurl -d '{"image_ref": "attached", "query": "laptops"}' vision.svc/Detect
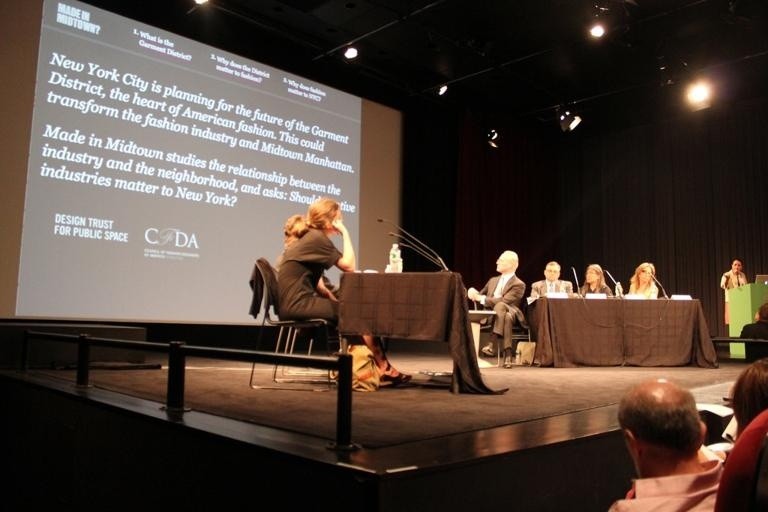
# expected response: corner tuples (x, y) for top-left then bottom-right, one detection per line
(754, 274), (768, 285)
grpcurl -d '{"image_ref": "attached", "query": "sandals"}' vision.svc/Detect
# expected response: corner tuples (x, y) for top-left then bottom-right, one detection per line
(380, 364), (412, 383)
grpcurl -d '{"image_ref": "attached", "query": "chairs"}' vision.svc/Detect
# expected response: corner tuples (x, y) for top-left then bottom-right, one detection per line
(714, 409), (768, 511)
(249, 257), (338, 392)
(512, 327), (531, 345)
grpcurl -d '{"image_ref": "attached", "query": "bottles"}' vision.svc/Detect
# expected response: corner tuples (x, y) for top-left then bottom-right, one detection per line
(615, 282), (623, 297)
(389, 243), (401, 272)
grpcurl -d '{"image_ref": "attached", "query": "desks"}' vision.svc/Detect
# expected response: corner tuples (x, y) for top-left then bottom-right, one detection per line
(528, 297), (718, 368)
(468, 311), (496, 368)
(339, 271), (509, 395)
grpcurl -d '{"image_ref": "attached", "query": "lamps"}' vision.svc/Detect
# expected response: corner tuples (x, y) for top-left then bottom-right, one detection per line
(488, 128), (499, 147)
(194, 0), (208, 7)
(555, 105), (581, 132)
(682, 76), (712, 111)
(588, 11), (606, 38)
(438, 83), (447, 95)
(345, 43), (357, 59)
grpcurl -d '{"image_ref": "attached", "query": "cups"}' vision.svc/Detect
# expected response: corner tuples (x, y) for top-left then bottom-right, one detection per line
(391, 258), (402, 272)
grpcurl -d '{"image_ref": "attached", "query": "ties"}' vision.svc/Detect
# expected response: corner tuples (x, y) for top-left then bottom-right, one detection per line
(493, 276), (506, 297)
(550, 283), (553, 293)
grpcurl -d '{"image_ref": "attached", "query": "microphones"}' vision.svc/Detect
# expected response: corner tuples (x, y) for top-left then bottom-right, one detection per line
(398, 242), (436, 264)
(375, 216), (439, 257)
(603, 270), (617, 284)
(387, 231), (440, 263)
(570, 266), (581, 294)
(735, 271), (741, 287)
(649, 273), (668, 300)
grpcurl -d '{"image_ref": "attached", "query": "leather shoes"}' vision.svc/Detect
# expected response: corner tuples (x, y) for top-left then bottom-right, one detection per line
(503, 356), (512, 370)
(481, 345), (494, 356)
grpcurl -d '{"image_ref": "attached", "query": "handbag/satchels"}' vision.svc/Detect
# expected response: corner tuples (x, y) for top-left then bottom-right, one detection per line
(329, 343), (379, 392)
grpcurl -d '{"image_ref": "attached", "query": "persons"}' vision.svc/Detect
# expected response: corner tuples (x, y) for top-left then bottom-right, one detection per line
(530, 261), (573, 298)
(467, 250), (527, 368)
(277, 198), (412, 385)
(281, 216), (340, 302)
(720, 258), (748, 325)
(722, 361), (768, 440)
(581, 264), (613, 298)
(629, 262), (659, 299)
(739, 304), (767, 360)
(608, 379), (726, 512)
